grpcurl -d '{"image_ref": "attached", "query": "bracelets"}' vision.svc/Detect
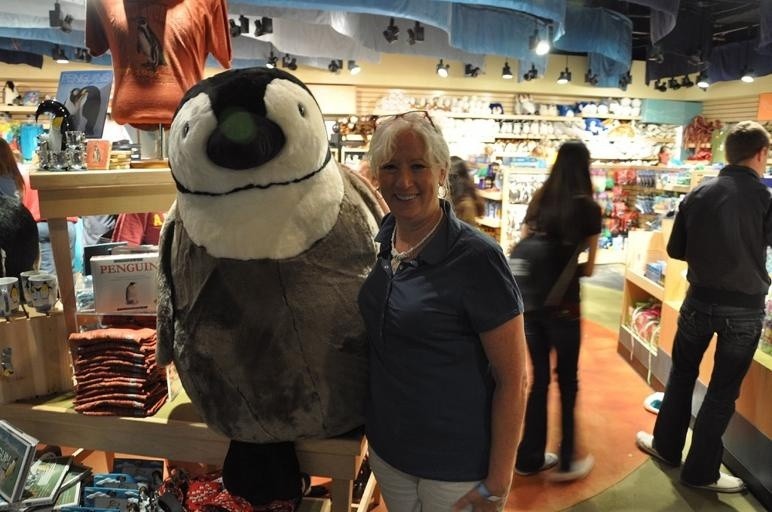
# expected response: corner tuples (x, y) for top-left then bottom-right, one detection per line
(476, 482), (508, 504)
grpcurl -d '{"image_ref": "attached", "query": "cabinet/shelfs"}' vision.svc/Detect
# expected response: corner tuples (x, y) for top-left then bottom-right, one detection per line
(0, 164), (377, 512)
(341, 112), (677, 166)
(1, 74), (116, 163)
(616, 219), (771, 512)
(591, 167), (719, 245)
(468, 162), (552, 255)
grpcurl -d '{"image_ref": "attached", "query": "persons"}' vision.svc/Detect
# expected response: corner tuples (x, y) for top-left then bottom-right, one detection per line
(85, 0), (234, 132)
(358, 112), (529, 512)
(514, 136), (600, 483)
(635, 120), (772, 495)
(1, 139), (163, 283)
(450, 156), (484, 227)
(652, 145), (681, 165)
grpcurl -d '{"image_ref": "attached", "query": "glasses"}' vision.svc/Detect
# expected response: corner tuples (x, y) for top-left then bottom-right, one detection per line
(374, 110), (437, 132)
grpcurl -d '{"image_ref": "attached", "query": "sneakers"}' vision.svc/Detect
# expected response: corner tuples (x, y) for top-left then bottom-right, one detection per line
(517, 450), (557, 477)
(550, 455), (596, 482)
(681, 469), (747, 492)
(637, 427), (680, 464)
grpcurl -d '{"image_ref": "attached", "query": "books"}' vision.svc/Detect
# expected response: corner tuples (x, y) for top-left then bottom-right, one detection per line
(0, 419), (93, 512)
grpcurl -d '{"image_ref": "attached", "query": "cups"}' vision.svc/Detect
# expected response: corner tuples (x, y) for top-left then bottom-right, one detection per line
(28, 274), (61, 313)
(20, 270), (48, 306)
(47, 130), (86, 171)
(85, 137), (111, 169)
(0, 277), (20, 317)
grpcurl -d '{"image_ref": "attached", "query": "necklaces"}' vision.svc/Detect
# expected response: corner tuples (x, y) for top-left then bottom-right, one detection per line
(389, 208), (446, 275)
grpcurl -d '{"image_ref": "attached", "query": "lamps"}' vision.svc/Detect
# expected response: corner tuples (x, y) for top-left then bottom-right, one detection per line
(738, 64), (756, 84)
(50, 2), (73, 34)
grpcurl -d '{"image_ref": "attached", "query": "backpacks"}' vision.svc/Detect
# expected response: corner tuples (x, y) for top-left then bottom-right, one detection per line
(506, 236), (569, 315)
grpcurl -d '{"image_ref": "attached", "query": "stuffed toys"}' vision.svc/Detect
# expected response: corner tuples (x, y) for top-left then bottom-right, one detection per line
(163, 65), (386, 502)
